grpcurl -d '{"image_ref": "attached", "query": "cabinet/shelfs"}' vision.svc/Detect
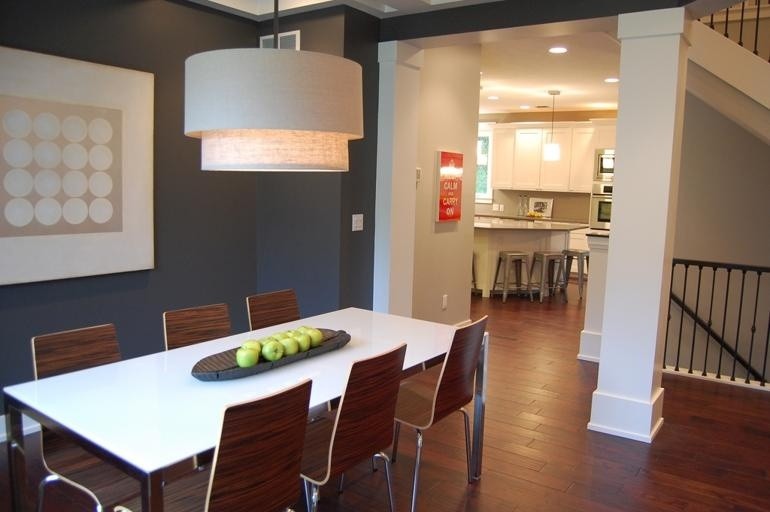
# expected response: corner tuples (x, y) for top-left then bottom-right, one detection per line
(513, 121), (571, 193)
(488, 123), (513, 191)
(570, 120), (599, 194)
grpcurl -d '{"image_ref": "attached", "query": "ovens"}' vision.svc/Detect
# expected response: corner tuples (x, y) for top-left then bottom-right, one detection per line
(590, 148), (616, 230)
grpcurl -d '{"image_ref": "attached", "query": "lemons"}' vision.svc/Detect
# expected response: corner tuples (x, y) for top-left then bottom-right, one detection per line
(527, 210), (542, 217)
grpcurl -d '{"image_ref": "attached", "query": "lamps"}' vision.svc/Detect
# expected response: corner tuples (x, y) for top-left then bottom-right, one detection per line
(183, 0), (365, 173)
(543, 90), (561, 161)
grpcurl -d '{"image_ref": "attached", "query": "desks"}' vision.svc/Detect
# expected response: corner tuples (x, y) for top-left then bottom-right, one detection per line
(1, 305), (494, 512)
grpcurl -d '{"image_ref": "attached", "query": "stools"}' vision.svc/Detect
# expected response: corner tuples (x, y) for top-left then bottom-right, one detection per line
(553, 247), (589, 301)
(470, 251), (478, 298)
(527, 250), (568, 305)
(490, 251), (534, 304)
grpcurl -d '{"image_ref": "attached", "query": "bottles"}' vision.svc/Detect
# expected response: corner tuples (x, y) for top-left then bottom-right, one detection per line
(516, 192), (529, 217)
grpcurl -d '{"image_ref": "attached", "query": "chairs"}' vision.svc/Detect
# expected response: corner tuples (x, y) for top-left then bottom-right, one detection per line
(113, 376), (313, 511)
(391, 315), (489, 511)
(31, 323), (179, 512)
(300, 342), (408, 512)
(243, 288), (336, 414)
(159, 301), (233, 471)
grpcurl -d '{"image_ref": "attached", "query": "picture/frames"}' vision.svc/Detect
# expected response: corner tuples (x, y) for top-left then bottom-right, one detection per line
(529, 197), (553, 217)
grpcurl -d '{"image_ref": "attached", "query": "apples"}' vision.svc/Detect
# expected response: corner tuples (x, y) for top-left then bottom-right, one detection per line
(236, 328), (323, 368)
